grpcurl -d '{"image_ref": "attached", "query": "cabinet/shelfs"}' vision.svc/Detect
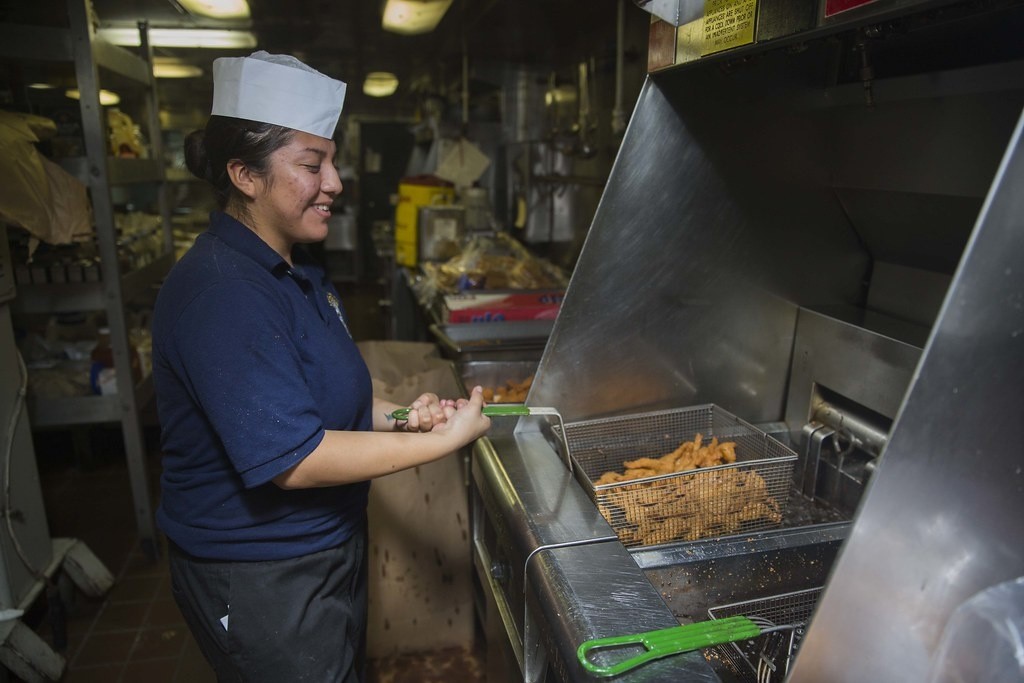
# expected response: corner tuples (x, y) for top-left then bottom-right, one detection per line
(1, 0), (175, 554)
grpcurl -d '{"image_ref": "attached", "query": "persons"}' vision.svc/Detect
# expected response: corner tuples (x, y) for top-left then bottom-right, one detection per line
(152, 50), (491, 683)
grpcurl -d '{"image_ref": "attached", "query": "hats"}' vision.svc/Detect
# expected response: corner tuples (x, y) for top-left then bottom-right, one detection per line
(210, 51), (347, 140)
